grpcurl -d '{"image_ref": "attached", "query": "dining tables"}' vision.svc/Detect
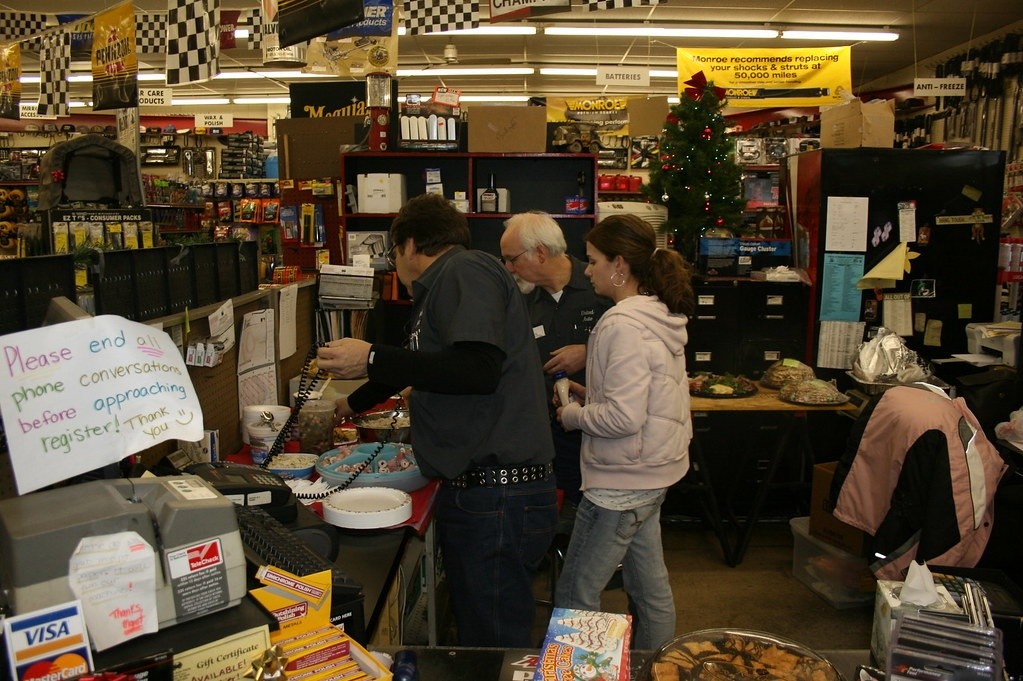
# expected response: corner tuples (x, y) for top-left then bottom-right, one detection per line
(689, 380), (857, 568)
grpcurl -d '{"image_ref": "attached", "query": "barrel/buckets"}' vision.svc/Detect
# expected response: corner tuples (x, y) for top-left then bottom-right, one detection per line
(247, 421), (285, 462)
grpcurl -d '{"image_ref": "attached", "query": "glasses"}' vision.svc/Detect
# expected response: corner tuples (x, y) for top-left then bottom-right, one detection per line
(498, 250), (527, 265)
(385, 244), (398, 267)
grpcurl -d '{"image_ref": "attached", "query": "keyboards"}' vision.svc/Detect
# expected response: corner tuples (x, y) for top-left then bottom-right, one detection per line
(231, 503), (363, 590)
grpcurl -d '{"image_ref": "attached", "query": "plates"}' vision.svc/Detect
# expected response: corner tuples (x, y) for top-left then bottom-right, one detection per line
(777, 393), (849, 406)
(321, 486), (412, 530)
(332, 427), (358, 446)
(759, 378), (781, 389)
(686, 374), (758, 399)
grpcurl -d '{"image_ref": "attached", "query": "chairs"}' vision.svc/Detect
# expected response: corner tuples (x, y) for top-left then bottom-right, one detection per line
(888, 387), (1023, 576)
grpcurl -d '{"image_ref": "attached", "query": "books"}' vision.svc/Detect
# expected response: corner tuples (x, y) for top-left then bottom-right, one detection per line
(317, 302), (368, 344)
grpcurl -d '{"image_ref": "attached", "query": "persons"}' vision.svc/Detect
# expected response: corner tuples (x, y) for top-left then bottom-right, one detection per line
(313, 196), (555, 652)
(556, 213), (697, 651)
(498, 209), (623, 586)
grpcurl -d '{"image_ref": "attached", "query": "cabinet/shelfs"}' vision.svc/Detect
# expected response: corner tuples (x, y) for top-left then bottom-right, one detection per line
(340, 149), (598, 266)
(683, 273), (810, 374)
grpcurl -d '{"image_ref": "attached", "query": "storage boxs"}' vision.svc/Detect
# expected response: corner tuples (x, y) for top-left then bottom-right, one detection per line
(357, 173), (407, 213)
(468, 105), (547, 152)
(788, 458), (878, 622)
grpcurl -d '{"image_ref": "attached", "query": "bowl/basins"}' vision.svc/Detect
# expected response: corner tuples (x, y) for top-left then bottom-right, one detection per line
(261, 453), (317, 480)
(350, 409), (409, 442)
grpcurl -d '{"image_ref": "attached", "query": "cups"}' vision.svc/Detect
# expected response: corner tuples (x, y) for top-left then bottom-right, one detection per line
(299, 400), (336, 455)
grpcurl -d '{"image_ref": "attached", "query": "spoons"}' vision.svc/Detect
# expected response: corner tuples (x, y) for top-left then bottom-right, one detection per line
(260, 410), (278, 432)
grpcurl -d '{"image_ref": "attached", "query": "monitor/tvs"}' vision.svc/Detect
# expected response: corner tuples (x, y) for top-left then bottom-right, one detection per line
(41, 296), (121, 483)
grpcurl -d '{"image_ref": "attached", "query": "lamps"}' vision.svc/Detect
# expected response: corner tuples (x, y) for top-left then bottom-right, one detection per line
(18, 24), (898, 113)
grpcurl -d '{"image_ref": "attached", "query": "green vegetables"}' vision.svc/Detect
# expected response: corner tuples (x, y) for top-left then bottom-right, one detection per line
(702, 370), (747, 394)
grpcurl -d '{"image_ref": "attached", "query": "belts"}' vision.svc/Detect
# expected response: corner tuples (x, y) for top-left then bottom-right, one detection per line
(442, 462), (553, 488)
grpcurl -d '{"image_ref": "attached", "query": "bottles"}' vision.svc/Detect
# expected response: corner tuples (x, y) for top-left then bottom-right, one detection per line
(293, 391), (323, 439)
(566, 172), (580, 214)
(555, 369), (574, 407)
(480, 171), (499, 213)
(577, 171), (588, 214)
